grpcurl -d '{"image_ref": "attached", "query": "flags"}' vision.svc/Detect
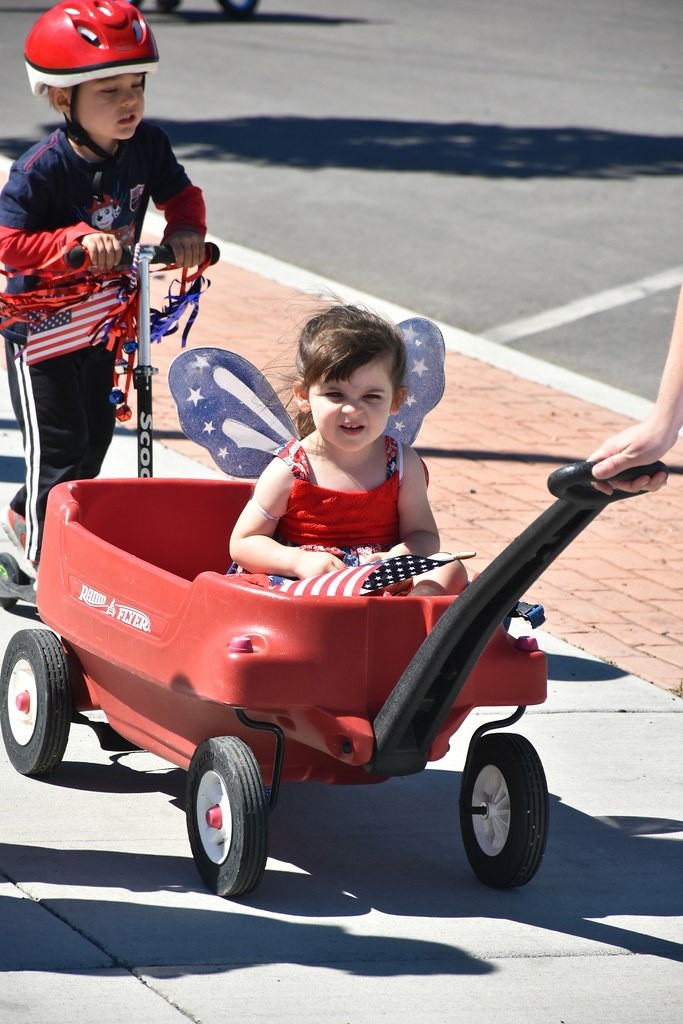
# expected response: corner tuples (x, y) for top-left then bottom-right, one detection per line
(267, 552), (476, 595)
(27, 275), (131, 365)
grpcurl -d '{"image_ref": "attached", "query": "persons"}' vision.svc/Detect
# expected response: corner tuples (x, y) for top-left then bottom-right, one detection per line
(0, 0), (207, 575)
(585, 282), (683, 496)
(230, 306), (468, 596)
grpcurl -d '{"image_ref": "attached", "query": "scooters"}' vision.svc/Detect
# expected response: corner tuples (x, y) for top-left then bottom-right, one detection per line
(1, 240), (222, 617)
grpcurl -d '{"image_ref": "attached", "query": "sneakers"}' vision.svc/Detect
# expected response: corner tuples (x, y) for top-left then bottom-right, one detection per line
(1, 504), (40, 573)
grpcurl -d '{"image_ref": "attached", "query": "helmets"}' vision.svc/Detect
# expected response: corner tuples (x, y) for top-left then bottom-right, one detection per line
(24, 0), (159, 96)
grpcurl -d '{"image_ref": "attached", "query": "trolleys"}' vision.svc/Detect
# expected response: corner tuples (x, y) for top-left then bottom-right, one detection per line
(0, 450), (666, 897)
